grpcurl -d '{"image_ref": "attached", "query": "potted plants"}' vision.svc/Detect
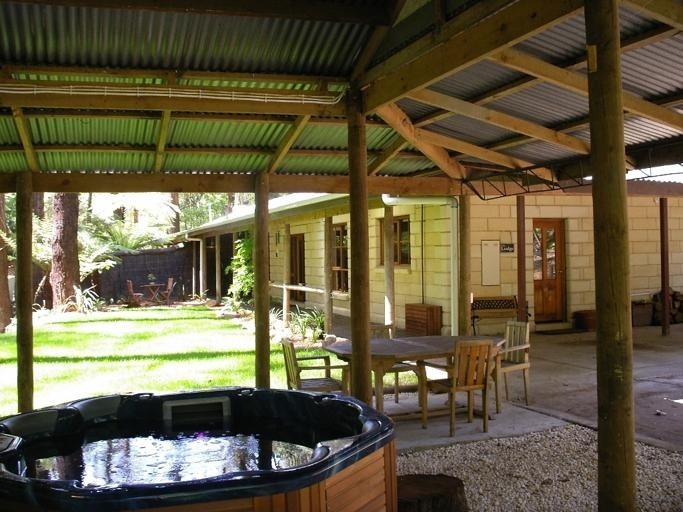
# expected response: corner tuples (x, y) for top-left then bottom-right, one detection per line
(144, 272), (158, 285)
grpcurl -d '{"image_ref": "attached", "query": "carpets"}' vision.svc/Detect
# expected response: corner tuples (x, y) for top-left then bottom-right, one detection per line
(535, 329), (588, 335)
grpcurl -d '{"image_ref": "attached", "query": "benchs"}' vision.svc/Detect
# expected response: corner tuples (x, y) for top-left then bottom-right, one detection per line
(471, 296), (531, 336)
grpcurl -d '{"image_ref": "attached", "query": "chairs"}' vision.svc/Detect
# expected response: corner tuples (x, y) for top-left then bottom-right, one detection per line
(282, 321), (530, 437)
(158, 278), (174, 304)
(126, 280), (145, 308)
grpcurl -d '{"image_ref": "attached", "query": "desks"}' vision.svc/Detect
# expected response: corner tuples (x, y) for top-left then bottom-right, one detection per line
(140, 284), (165, 306)
